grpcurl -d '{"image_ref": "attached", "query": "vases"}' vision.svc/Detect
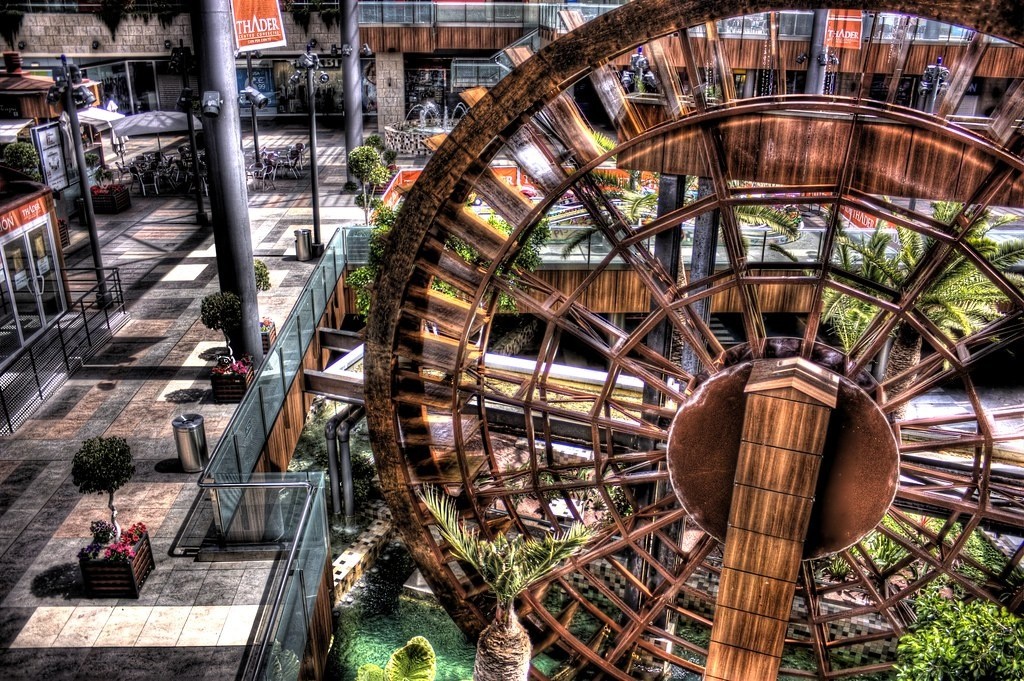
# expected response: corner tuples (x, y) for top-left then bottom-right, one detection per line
(262, 323), (277, 355)
(78, 531), (156, 599)
(92, 187), (131, 215)
(212, 369), (254, 402)
(59, 228), (71, 248)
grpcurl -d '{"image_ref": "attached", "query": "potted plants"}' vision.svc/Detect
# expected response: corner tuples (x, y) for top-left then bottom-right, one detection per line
(342, 146), (392, 261)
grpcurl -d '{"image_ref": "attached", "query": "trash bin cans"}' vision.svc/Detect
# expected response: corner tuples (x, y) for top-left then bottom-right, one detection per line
(294, 229), (312, 261)
(172, 414), (210, 473)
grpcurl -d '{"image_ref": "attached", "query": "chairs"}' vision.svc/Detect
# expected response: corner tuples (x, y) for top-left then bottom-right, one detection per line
(115, 139), (307, 197)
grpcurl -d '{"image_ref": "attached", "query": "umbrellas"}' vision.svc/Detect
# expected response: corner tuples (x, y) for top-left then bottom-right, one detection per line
(110, 109), (203, 164)
(59, 110), (78, 184)
(106, 100), (130, 167)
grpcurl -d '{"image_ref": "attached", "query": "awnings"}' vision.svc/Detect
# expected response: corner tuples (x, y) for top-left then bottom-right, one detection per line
(0, 117), (34, 144)
(77, 106), (125, 132)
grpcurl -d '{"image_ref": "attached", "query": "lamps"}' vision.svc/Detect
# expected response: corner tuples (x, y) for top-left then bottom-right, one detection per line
(92, 39), (100, 51)
(916, 62), (953, 99)
(202, 92), (223, 118)
(342, 44), (352, 57)
(44, 65), (96, 116)
(289, 51), (331, 90)
(817, 52), (829, 67)
(177, 87), (201, 113)
(623, 50), (660, 92)
(387, 76), (392, 88)
(796, 52), (810, 64)
(331, 44), (341, 56)
(360, 42), (372, 56)
(237, 87), (270, 110)
(16, 40), (27, 50)
(309, 38), (317, 48)
(828, 54), (839, 66)
(165, 39), (172, 49)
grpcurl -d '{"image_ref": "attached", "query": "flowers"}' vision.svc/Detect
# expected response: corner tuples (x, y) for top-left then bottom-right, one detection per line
(200, 292), (250, 377)
(57, 217), (68, 227)
(85, 154), (126, 195)
(71, 434), (148, 560)
(253, 258), (272, 333)
